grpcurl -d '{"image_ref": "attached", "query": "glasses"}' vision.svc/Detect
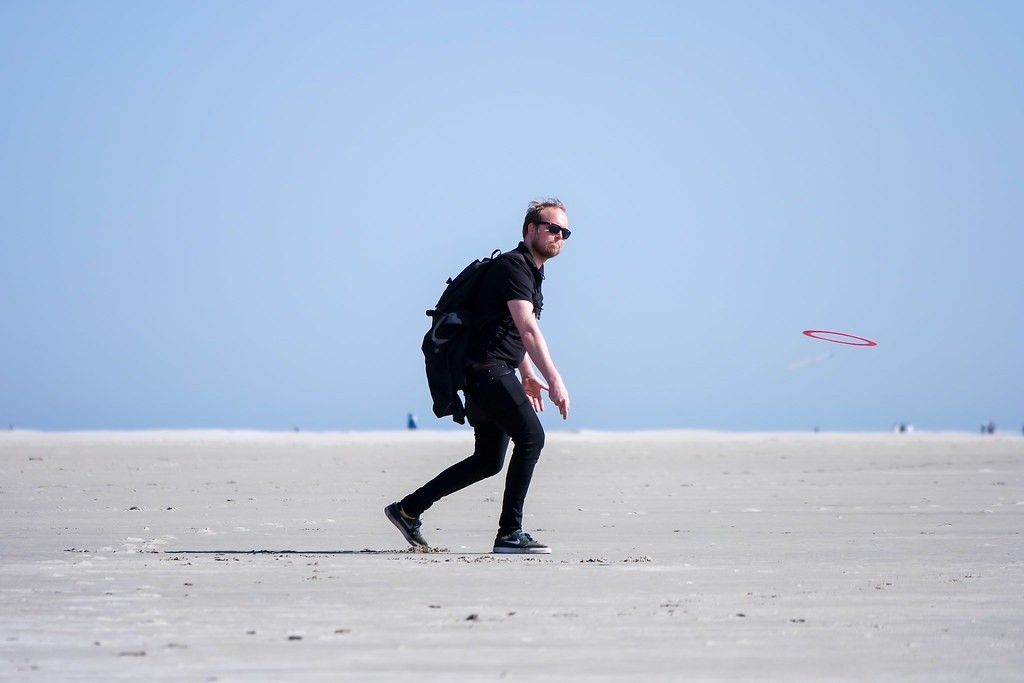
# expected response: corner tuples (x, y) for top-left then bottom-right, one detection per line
(534, 221), (571, 239)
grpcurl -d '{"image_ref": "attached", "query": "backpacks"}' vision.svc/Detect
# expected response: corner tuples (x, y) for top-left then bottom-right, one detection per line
(425, 250), (527, 360)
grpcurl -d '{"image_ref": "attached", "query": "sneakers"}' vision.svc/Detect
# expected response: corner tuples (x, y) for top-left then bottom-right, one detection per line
(384, 502), (428, 547)
(493, 529), (551, 554)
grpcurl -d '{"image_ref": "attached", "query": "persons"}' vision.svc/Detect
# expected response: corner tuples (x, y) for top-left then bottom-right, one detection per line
(384, 199), (570, 553)
